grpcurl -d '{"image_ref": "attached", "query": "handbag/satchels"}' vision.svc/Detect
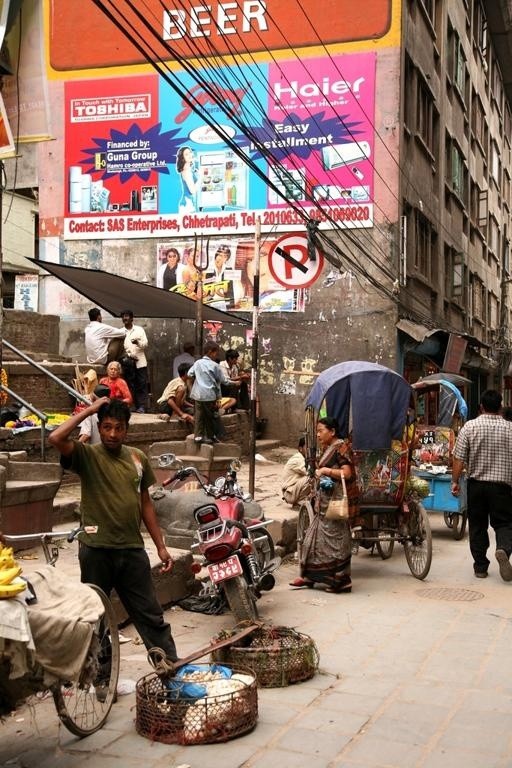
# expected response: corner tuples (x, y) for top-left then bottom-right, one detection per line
(326, 497), (350, 521)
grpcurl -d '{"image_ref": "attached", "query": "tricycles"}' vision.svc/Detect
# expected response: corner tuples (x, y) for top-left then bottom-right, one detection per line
(295, 360), (434, 583)
(409, 371), (474, 541)
(0, 526), (121, 739)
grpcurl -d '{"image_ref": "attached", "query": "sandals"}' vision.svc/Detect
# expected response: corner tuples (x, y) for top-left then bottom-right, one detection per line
(205, 437), (218, 444)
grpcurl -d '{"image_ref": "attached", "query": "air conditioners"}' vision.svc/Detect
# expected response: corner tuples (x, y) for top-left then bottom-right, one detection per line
(320, 140), (373, 170)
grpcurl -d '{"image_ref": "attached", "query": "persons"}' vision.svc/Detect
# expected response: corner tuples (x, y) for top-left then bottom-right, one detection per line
(96, 360), (135, 409)
(156, 339), (251, 444)
(181, 247), (200, 275)
(84, 307), (126, 363)
(288, 418), (359, 593)
(77, 384), (112, 448)
(161, 247), (189, 292)
(450, 389), (511, 582)
(235, 242), (295, 310)
(500, 407), (511, 423)
(118, 310), (149, 412)
(47, 397), (178, 702)
(282, 436), (311, 512)
(202, 245), (235, 308)
(176, 144), (204, 215)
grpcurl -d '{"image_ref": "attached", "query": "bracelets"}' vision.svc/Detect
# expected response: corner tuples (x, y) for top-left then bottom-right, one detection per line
(329, 466), (332, 476)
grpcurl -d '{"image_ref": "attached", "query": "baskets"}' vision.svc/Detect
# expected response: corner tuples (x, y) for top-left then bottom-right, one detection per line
(207, 624), (321, 686)
(135, 662), (258, 744)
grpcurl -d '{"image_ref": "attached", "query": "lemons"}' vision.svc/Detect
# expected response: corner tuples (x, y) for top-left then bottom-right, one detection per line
(5, 421), (16, 428)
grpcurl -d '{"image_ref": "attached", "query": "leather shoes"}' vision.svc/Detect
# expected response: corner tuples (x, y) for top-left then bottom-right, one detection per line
(137, 407), (146, 412)
(194, 437), (204, 443)
(496, 550), (512, 582)
(476, 572), (488, 578)
(130, 406), (139, 412)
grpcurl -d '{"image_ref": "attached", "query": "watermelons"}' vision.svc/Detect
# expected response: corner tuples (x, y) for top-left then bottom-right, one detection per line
(406, 475), (429, 497)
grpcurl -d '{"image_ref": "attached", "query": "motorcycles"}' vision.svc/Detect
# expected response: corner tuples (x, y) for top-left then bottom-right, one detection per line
(160, 457), (276, 630)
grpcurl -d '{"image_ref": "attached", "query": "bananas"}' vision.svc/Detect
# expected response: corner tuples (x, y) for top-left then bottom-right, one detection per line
(0, 543), (27, 599)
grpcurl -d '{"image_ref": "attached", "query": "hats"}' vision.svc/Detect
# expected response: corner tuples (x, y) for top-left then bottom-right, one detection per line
(94, 384), (110, 399)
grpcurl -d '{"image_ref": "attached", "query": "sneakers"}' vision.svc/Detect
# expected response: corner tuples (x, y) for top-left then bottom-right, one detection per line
(95, 680), (120, 703)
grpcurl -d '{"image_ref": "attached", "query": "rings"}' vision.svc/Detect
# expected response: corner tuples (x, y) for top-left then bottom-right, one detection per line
(162, 562), (167, 566)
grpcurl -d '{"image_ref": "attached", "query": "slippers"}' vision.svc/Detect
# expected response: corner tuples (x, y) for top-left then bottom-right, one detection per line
(289, 577), (316, 588)
(327, 584), (354, 594)
